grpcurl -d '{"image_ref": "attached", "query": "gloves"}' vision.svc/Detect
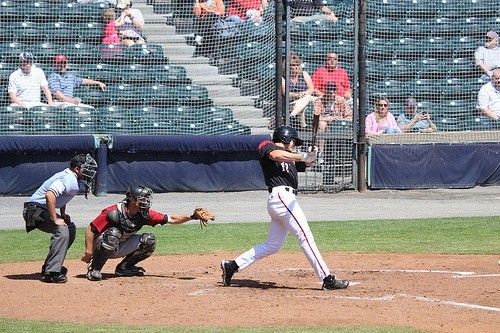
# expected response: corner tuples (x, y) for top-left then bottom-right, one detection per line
(300, 150), (317, 162)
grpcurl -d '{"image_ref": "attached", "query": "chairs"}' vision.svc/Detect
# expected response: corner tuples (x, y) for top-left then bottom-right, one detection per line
(1, 0), (251, 137)
(237, 0), (500, 130)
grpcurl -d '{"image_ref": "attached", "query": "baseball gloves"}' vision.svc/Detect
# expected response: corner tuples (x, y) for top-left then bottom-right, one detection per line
(194, 206), (215, 224)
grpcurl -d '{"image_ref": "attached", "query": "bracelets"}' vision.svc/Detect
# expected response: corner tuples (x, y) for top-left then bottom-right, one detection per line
(130, 16), (134, 20)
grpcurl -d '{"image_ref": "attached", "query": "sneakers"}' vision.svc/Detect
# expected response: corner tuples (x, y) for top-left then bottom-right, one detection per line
(322, 276), (349, 290)
(87, 268), (103, 281)
(221, 260), (234, 286)
(115, 266), (146, 275)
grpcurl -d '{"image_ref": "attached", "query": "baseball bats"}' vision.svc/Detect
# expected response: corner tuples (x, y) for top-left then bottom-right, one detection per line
(311, 112), (320, 151)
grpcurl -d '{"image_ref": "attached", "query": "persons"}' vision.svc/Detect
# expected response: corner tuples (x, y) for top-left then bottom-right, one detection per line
(8, 52), (107, 129)
(290, 0), (335, 22)
(474, 31), (500, 82)
(192, 0), (269, 25)
(365, 97), (437, 136)
(22, 153), (98, 284)
(220, 125), (349, 290)
(475, 68), (500, 131)
(281, 51), (352, 166)
(98, 0), (150, 59)
(80, 186), (215, 281)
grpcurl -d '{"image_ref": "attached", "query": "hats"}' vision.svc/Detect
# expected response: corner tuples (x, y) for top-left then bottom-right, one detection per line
(404, 98), (416, 108)
(56, 55), (65, 62)
(70, 155), (94, 167)
(487, 31), (498, 40)
(19, 52), (33, 61)
(117, 0), (131, 8)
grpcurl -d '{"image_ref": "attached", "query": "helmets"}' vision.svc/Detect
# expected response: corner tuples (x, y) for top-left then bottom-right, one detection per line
(273, 126), (304, 146)
(122, 185), (148, 201)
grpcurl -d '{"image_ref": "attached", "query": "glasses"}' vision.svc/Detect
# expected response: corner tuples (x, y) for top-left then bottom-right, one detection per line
(377, 104), (388, 107)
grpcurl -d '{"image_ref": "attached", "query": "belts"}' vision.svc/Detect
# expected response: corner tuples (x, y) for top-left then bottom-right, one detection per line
(269, 186), (297, 196)
(23, 203), (39, 208)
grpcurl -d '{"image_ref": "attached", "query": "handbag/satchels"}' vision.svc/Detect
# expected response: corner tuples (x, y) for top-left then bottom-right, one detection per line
(25, 204), (52, 232)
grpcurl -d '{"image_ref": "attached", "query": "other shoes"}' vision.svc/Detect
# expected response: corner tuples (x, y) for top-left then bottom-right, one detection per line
(43, 265), (68, 274)
(317, 153), (325, 165)
(290, 116), (297, 126)
(41, 271), (68, 282)
